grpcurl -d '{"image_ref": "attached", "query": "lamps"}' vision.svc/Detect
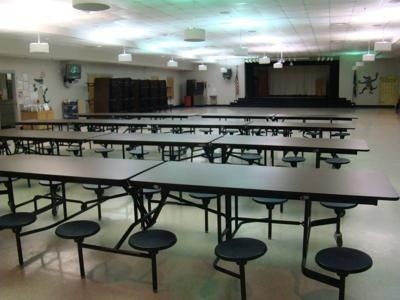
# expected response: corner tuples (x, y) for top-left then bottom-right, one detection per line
(356, 28), (392, 67)
(118, 49), (133, 62)
(29, 30), (49, 55)
(184, 26), (206, 41)
(167, 57), (209, 71)
(259, 49), (283, 69)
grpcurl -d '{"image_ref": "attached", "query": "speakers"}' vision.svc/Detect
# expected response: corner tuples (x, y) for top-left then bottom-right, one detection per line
(223, 69), (231, 78)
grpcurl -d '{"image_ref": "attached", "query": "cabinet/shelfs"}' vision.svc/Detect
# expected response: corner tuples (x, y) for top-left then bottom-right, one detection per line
(96, 79), (168, 114)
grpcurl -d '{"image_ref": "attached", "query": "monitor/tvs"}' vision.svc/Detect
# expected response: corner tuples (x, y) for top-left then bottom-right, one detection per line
(66, 63), (81, 79)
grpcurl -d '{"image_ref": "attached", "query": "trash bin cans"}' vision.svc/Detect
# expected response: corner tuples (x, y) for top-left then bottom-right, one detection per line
(184, 95), (191, 107)
